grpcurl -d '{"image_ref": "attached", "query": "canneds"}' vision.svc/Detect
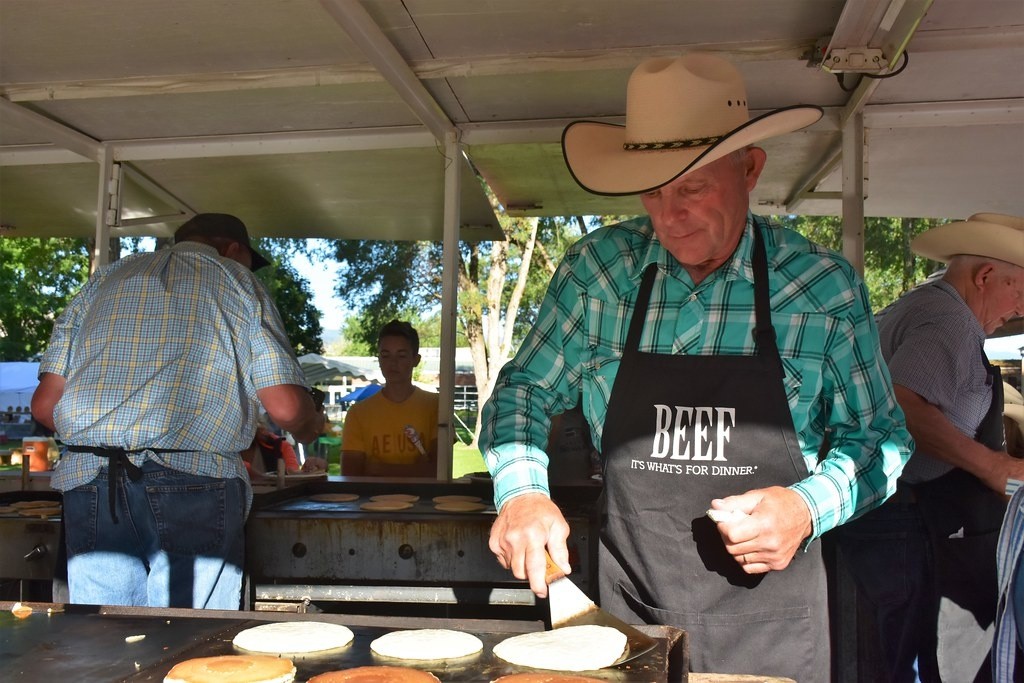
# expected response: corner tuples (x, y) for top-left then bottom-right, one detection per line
(22, 436), (50, 473)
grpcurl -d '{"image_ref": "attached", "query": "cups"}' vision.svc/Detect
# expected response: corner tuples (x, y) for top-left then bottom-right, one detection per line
(22, 436), (49, 472)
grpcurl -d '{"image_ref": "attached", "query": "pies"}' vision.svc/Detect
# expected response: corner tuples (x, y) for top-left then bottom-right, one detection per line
(307, 489), (486, 510)
(162, 619), (630, 683)
(0, 499), (61, 516)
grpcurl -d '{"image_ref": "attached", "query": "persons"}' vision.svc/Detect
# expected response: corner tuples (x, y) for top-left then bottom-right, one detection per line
(340, 319), (459, 478)
(836, 212), (1024, 683)
(477, 53), (917, 683)
(30, 212), (326, 612)
(239, 412), (300, 476)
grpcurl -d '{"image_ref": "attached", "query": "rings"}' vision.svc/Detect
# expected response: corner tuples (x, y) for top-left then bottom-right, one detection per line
(743, 554), (748, 563)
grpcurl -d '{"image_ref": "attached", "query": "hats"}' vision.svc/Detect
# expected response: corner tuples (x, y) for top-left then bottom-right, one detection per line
(560, 52), (824, 196)
(173, 212), (271, 272)
(910, 213), (1024, 268)
